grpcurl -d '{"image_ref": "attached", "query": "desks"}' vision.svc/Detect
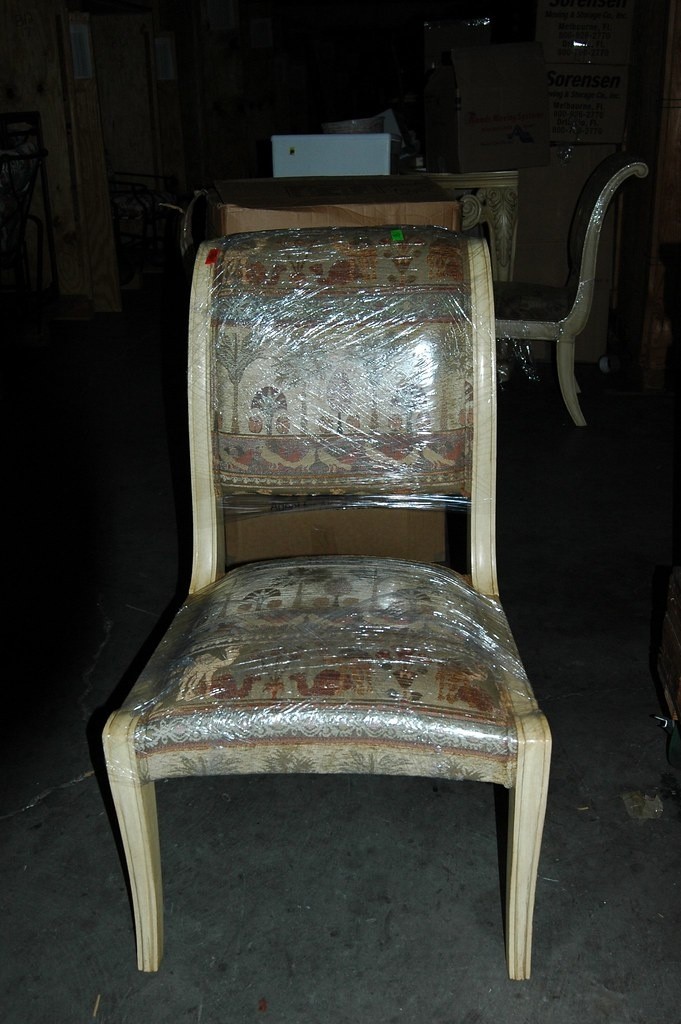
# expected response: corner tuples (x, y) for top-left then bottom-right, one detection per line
(174, 168), (520, 286)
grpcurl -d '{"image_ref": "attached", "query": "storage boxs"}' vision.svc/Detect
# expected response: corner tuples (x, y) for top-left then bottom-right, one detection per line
(204, 174), (465, 240)
(271, 134), (402, 176)
(417, 0), (637, 366)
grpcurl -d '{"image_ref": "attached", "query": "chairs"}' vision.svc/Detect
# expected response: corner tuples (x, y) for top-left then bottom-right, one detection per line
(100, 216), (553, 982)
(0, 110), (61, 337)
(102, 147), (178, 291)
(490, 149), (650, 428)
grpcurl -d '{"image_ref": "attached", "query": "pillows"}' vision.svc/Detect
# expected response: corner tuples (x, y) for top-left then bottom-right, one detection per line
(113, 190), (188, 217)
